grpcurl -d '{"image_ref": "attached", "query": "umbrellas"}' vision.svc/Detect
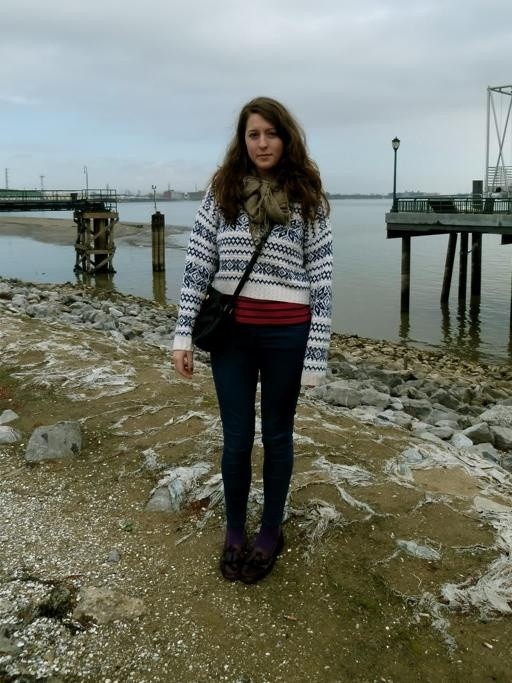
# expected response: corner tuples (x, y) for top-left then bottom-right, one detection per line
(219, 542), (245, 580)
(239, 529), (284, 584)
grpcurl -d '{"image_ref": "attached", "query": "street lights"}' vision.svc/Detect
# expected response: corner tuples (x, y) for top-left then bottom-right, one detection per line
(192, 285), (234, 352)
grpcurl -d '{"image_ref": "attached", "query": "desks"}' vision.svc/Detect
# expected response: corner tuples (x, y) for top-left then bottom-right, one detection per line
(390, 135), (400, 212)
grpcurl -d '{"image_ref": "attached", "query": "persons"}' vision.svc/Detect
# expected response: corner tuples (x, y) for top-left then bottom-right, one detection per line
(173, 96), (334, 584)
